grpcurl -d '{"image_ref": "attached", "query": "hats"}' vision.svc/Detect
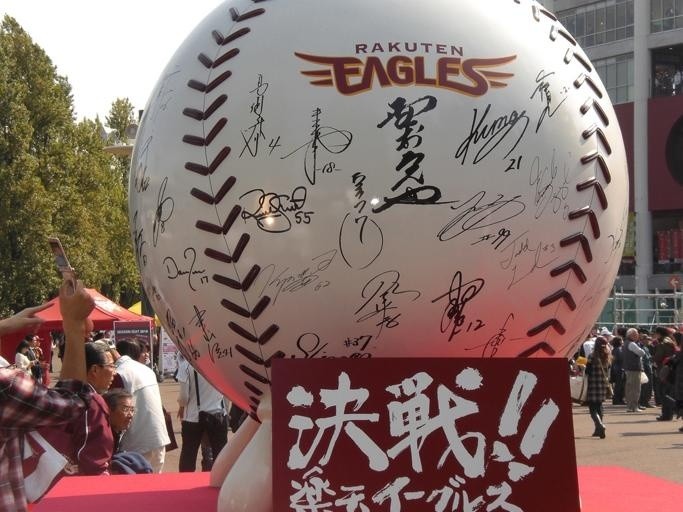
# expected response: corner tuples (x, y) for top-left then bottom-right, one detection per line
(34, 335), (43, 341)
(601, 329), (612, 336)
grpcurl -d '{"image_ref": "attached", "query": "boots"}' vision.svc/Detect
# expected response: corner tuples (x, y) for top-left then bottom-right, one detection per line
(592, 413), (606, 438)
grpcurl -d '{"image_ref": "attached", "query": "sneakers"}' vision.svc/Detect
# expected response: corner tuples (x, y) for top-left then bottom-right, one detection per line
(612, 398), (656, 412)
(656, 415), (673, 421)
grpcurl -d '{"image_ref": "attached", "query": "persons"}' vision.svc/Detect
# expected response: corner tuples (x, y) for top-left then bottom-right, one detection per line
(569, 325), (682, 440)
(0, 273), (252, 512)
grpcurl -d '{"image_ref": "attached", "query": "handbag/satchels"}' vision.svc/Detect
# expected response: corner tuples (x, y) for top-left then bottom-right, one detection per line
(31, 364), (42, 378)
(164, 412), (178, 452)
(571, 376), (588, 402)
(610, 364), (618, 383)
(42, 362), (51, 386)
(197, 411), (227, 448)
(641, 372), (649, 384)
(606, 384), (614, 398)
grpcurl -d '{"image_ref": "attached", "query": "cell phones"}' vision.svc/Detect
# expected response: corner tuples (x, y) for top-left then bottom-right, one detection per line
(47, 237), (77, 296)
